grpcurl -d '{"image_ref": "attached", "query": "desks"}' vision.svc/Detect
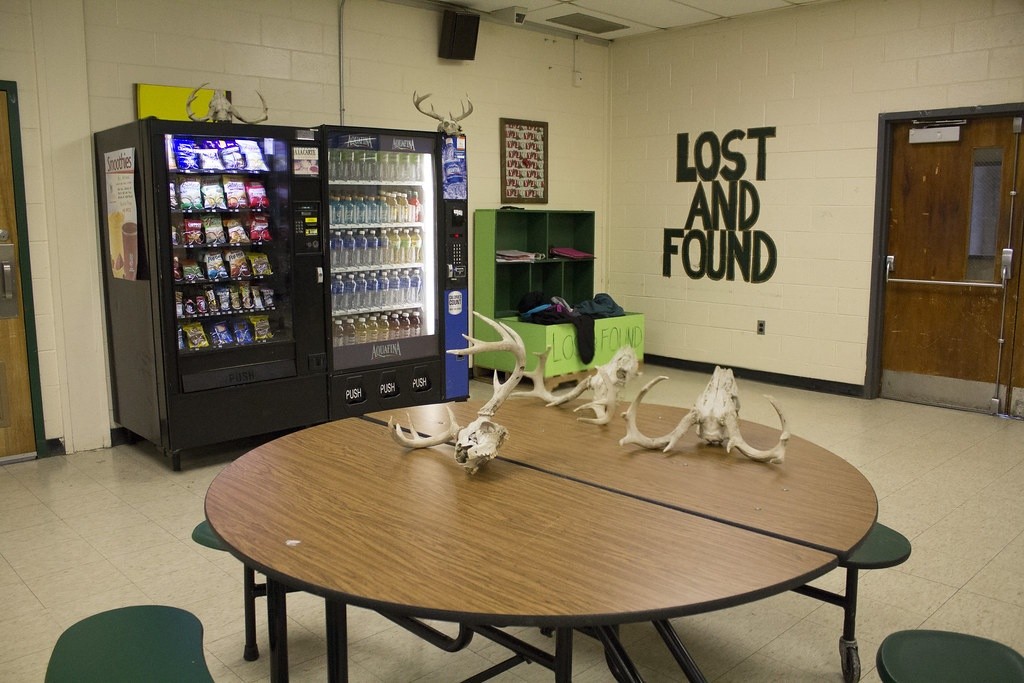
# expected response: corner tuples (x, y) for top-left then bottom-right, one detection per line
(205, 396), (878, 682)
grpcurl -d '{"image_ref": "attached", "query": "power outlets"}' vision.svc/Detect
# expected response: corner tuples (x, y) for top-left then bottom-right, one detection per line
(757, 320), (765, 335)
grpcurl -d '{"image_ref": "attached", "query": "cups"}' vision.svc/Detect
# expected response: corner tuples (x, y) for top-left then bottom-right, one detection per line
(122, 222), (137, 277)
(108, 211), (125, 278)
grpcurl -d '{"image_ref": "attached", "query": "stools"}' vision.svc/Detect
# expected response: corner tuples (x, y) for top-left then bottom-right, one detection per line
(876, 629), (1024, 683)
(192, 520), (267, 662)
(44, 606), (215, 683)
(838, 523), (911, 683)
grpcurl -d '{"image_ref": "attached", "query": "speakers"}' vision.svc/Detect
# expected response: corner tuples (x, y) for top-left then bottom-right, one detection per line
(438, 9), (480, 60)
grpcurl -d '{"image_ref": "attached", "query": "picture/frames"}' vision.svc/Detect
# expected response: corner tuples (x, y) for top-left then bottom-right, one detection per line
(500, 118), (548, 203)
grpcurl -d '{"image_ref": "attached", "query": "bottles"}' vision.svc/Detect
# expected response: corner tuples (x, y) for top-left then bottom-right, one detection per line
(327, 148), (424, 180)
(329, 189), (420, 224)
(331, 269), (423, 310)
(332, 308), (422, 348)
(330, 227), (422, 266)
(445, 138), (466, 199)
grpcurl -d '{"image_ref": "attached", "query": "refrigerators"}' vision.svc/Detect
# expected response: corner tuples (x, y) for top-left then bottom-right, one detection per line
(93, 115), (471, 470)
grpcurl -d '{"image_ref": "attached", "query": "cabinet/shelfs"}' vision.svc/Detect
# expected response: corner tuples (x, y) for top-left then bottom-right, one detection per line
(473, 209), (644, 392)
